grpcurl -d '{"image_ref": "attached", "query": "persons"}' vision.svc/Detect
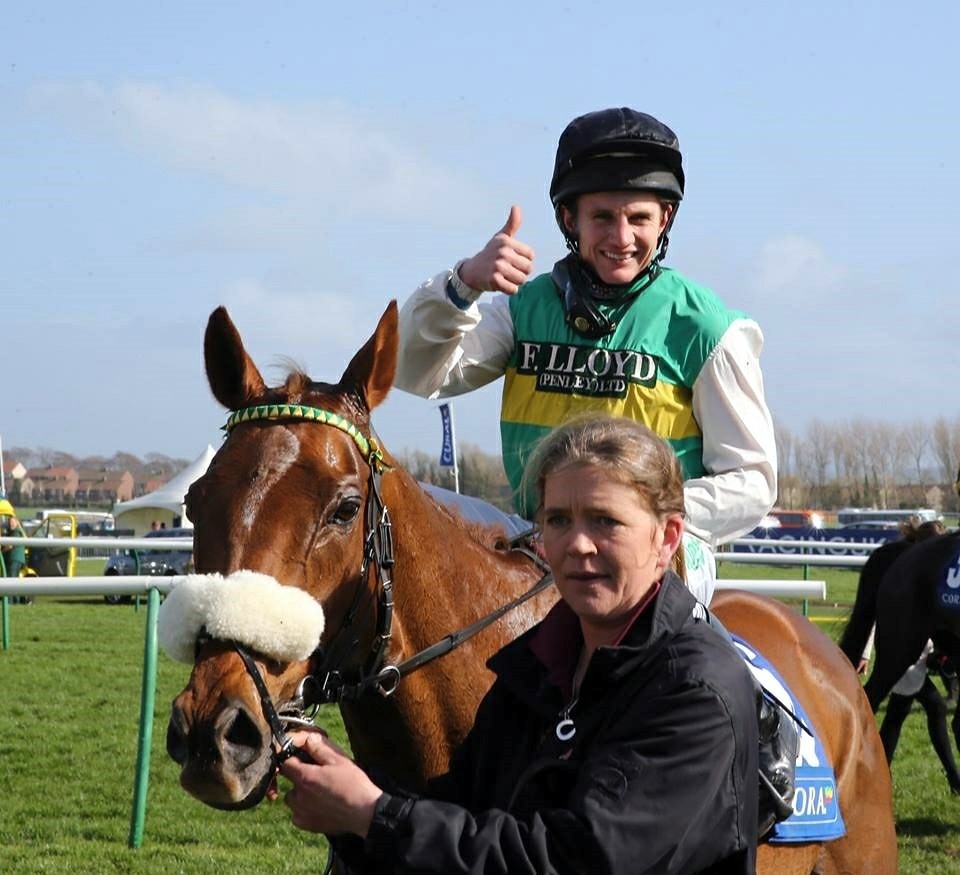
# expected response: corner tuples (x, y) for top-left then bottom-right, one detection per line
(270, 412), (761, 874)
(272, 108), (783, 875)
(150, 521), (167, 531)
(3, 516), (26, 604)
(855, 518), (960, 798)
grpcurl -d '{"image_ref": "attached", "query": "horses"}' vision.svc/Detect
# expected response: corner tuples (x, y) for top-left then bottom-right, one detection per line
(835, 517), (960, 801)
(147, 295), (904, 875)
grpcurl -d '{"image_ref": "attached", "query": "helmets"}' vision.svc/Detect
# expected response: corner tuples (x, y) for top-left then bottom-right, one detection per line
(551, 154), (684, 204)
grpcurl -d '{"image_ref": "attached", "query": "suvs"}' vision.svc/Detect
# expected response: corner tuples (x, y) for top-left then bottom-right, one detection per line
(103, 527), (195, 604)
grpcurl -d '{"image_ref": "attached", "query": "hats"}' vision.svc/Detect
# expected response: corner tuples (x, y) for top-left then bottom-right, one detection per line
(550, 108), (685, 195)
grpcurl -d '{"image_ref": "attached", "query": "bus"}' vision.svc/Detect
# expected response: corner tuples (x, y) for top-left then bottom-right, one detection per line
(766, 507), (939, 530)
(36, 512), (115, 530)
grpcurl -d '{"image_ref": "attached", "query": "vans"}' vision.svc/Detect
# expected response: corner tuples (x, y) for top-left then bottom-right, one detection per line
(0, 499), (79, 579)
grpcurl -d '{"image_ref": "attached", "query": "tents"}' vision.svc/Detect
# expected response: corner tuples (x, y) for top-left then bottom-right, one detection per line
(113, 445), (216, 559)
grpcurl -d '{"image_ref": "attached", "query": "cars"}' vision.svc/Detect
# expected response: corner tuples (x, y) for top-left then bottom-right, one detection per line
(842, 521), (901, 532)
